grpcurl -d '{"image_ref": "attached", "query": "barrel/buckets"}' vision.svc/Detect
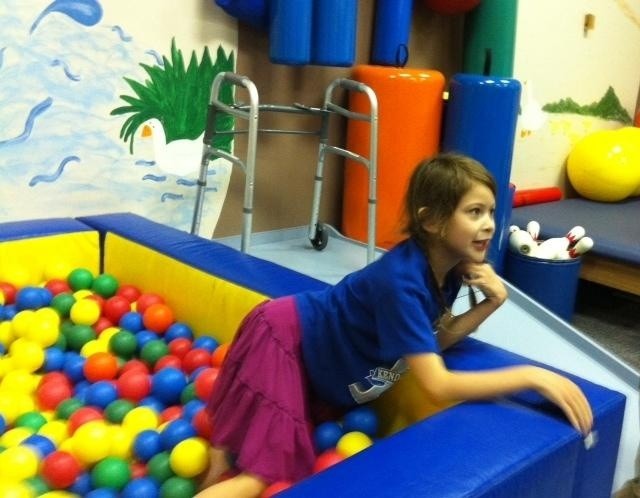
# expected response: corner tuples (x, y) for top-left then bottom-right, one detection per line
(507, 240), (581, 322)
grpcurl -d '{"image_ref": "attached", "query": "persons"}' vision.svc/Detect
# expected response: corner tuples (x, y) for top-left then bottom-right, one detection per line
(189, 151), (594, 497)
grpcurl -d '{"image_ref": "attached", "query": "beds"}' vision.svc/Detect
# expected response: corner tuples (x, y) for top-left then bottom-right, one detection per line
(510, 197), (640, 296)
